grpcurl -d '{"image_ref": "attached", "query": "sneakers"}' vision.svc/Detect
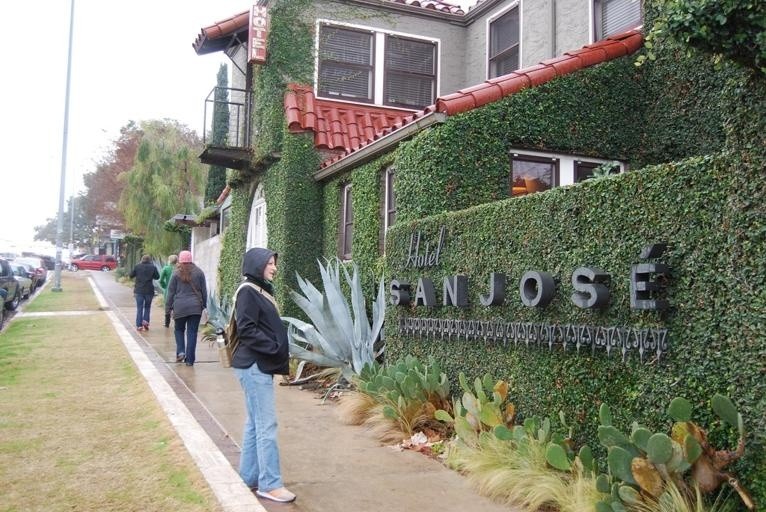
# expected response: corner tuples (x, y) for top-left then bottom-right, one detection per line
(141, 321), (148, 330)
(256, 487), (295, 503)
(136, 328), (142, 331)
(176, 356), (184, 361)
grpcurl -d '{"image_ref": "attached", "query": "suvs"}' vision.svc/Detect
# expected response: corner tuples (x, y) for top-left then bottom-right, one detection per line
(69, 253), (116, 272)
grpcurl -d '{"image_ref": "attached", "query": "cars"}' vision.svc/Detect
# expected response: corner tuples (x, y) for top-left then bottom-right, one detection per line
(0, 252), (68, 328)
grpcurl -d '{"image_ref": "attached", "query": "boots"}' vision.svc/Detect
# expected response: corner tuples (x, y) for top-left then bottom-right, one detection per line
(162, 314), (169, 327)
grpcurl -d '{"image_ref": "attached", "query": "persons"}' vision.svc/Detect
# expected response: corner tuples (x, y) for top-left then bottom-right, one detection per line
(129, 254), (160, 332)
(231, 246), (298, 504)
(165, 250), (207, 367)
(158, 255), (179, 328)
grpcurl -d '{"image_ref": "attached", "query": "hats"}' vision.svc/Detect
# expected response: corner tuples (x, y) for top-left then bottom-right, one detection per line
(178, 251), (191, 263)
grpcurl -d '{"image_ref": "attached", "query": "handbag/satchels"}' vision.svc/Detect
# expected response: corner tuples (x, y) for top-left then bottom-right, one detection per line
(217, 320), (239, 368)
(199, 308), (208, 324)
